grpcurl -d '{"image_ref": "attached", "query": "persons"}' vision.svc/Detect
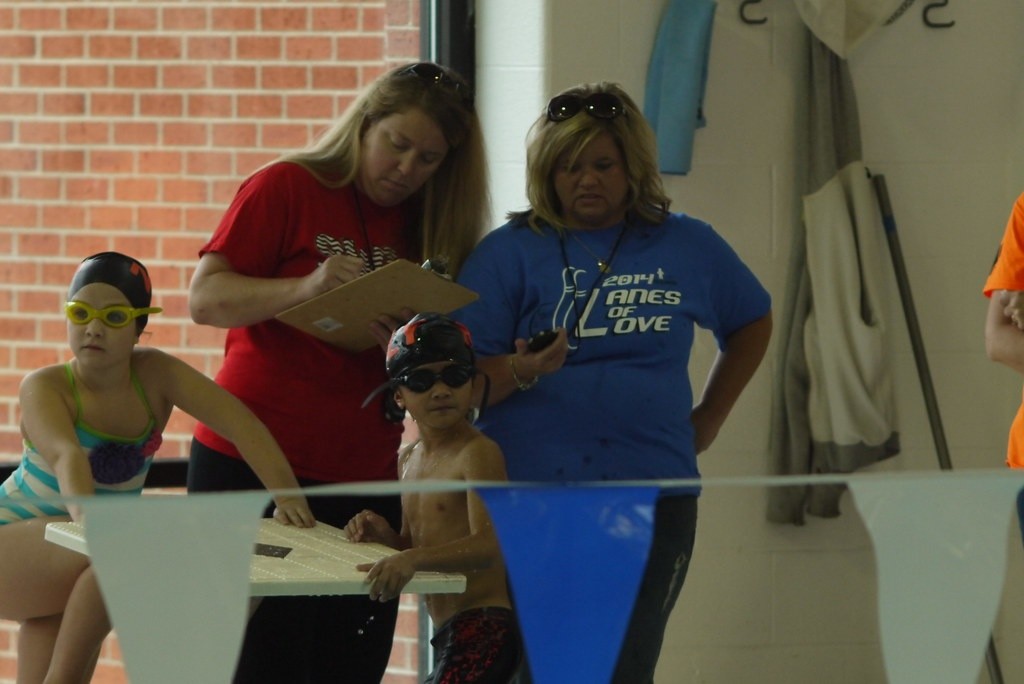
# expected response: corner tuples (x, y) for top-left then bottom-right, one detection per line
(0, 251), (315, 684)
(187, 61), (487, 684)
(448, 81), (775, 684)
(344, 312), (525, 684)
(982, 190), (1024, 545)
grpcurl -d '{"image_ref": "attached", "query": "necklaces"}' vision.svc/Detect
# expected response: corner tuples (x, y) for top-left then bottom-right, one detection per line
(565, 225), (629, 275)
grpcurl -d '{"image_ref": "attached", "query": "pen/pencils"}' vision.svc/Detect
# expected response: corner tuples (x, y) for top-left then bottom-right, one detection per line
(318, 262), (323, 267)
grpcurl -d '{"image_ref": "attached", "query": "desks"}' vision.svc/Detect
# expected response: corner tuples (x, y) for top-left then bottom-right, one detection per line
(45, 519), (466, 684)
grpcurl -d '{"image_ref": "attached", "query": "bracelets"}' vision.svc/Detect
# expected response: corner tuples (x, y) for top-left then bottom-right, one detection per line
(508, 353), (539, 391)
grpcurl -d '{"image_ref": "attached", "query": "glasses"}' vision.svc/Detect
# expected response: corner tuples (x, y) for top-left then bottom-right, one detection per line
(545, 90), (627, 120)
(391, 360), (477, 393)
(390, 62), (475, 109)
(66, 297), (163, 327)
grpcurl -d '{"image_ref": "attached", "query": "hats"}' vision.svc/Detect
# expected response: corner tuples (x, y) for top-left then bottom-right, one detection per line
(66, 249), (154, 331)
(384, 310), (479, 383)
